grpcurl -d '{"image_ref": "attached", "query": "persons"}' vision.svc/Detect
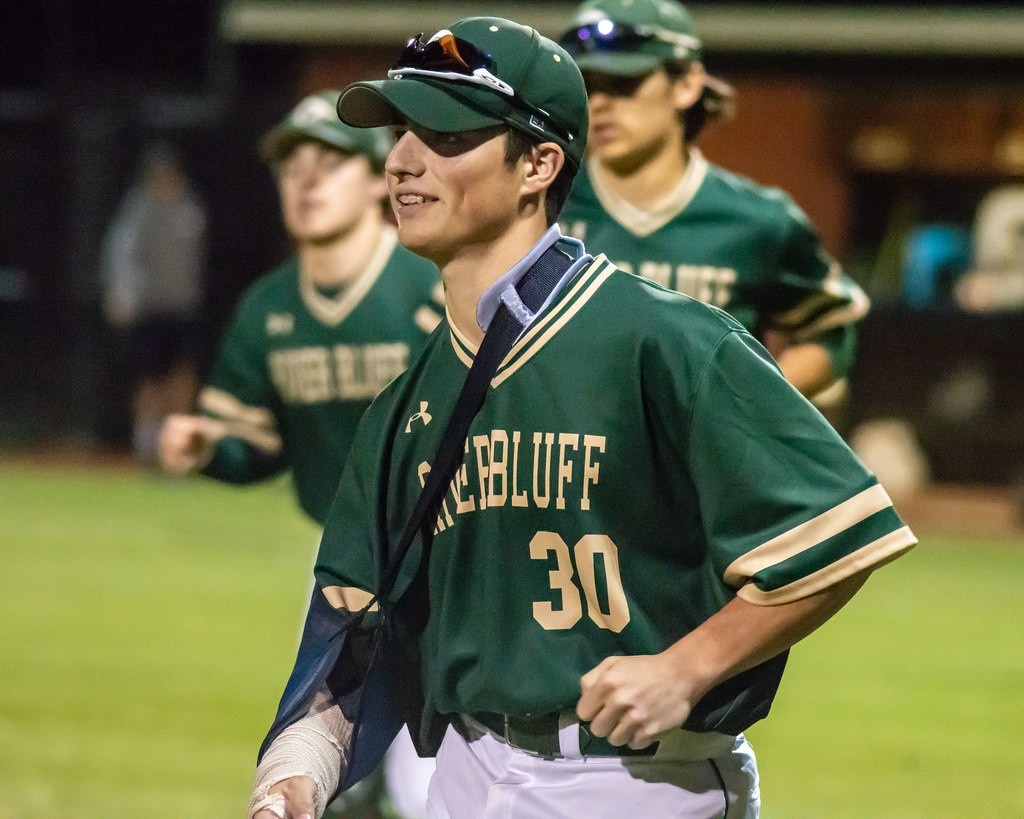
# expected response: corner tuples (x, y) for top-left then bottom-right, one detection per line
(97, 129), (214, 476)
(157, 0), (923, 819)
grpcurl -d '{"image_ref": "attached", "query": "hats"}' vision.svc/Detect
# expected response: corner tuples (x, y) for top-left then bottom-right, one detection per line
(259, 89), (398, 167)
(336, 17), (590, 172)
(557, 0), (703, 79)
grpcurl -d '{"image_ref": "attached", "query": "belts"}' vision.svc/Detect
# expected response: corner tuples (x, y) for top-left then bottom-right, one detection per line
(468, 707), (661, 758)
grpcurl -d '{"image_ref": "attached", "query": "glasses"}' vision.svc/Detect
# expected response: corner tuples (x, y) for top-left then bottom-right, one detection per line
(559, 21), (700, 56)
(388, 30), (574, 142)
